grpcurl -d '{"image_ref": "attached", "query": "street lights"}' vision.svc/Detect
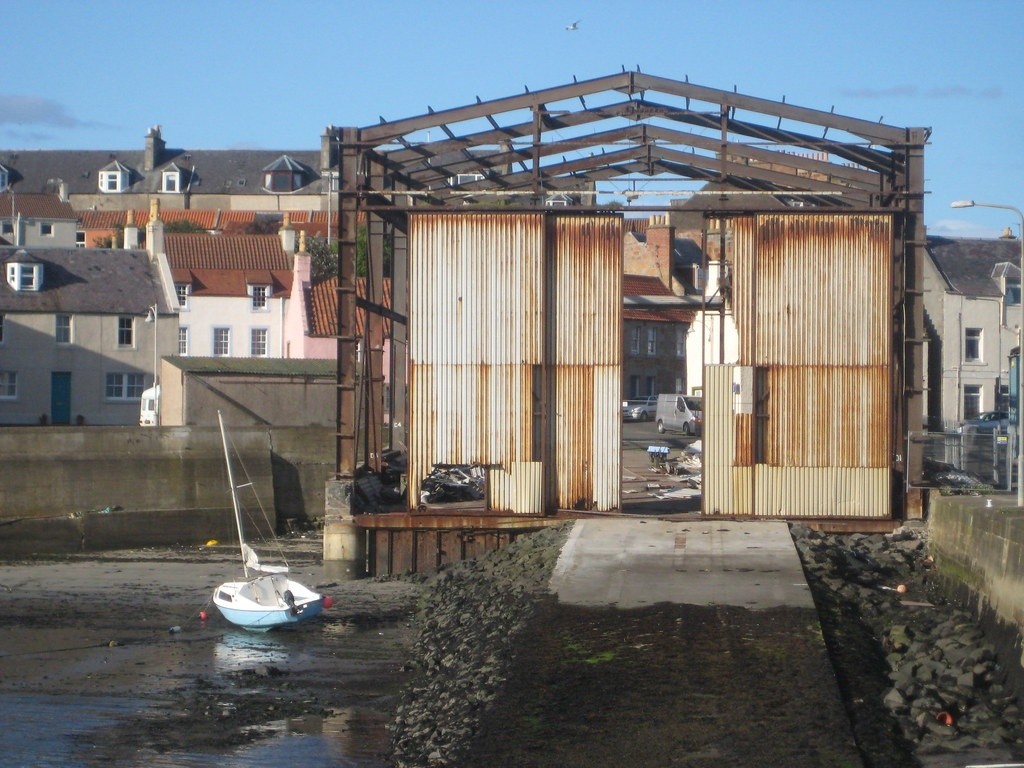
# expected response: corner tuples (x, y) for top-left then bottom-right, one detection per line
(949, 200), (1023, 508)
(145, 302), (158, 405)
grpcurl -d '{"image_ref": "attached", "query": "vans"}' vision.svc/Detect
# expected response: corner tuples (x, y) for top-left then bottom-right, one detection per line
(655, 393), (703, 436)
(140, 386), (161, 428)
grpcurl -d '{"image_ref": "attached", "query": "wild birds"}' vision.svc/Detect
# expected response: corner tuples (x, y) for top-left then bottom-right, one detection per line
(564, 19), (581, 31)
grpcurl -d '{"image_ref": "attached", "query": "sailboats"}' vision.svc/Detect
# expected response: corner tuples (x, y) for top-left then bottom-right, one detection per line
(211, 409), (327, 634)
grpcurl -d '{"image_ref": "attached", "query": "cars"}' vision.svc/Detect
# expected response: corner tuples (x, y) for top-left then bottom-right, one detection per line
(622, 395), (659, 423)
(958, 409), (1009, 434)
